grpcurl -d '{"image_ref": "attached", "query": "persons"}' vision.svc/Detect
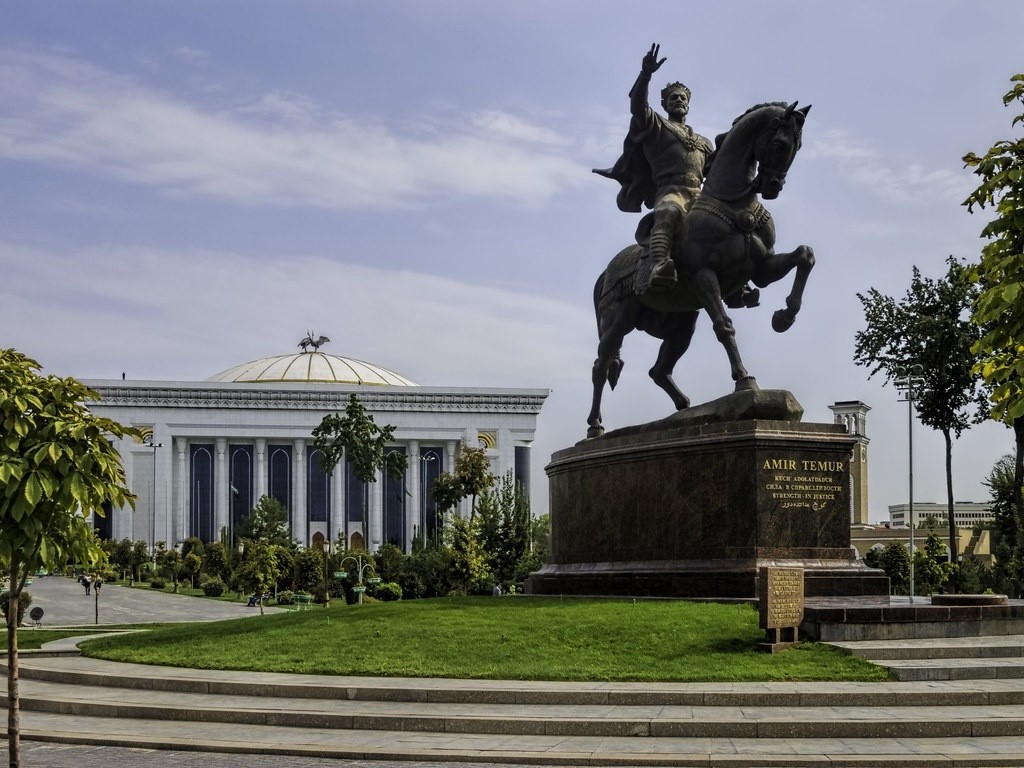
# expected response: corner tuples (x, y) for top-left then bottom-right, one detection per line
(92, 577), (102, 595)
(82, 573), (92, 595)
(591, 42), (760, 309)
(77, 572), (86, 582)
(493, 583), (501, 595)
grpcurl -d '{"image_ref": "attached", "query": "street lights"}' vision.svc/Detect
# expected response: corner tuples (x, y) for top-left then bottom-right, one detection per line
(172, 543), (180, 592)
(239, 542), (245, 597)
(323, 539), (331, 608)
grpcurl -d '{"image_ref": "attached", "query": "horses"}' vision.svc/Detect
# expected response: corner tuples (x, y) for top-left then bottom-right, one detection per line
(586, 99), (818, 441)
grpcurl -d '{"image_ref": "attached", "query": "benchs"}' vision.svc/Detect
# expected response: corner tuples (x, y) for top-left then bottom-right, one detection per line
(248, 593), (269, 606)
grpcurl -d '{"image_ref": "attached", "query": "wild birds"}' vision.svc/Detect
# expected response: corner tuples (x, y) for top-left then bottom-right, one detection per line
(296, 327), (331, 353)
